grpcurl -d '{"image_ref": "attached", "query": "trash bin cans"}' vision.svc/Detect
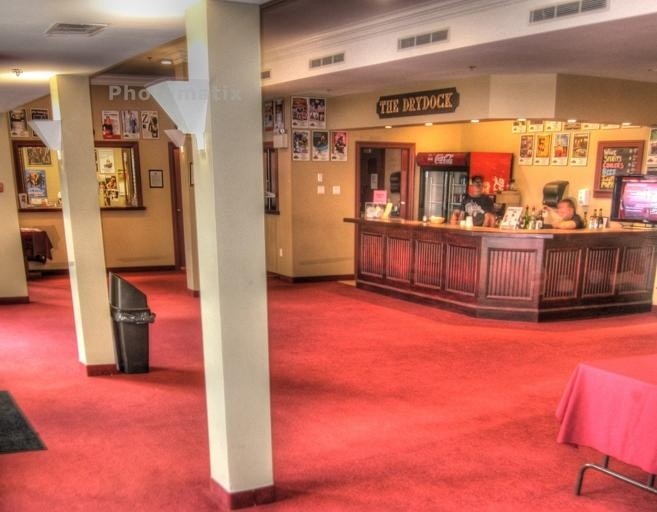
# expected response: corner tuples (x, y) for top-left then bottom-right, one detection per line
(109, 271), (150, 373)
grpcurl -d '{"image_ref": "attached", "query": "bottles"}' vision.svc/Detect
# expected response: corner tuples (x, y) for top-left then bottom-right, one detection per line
(523, 206), (543, 231)
(588, 208), (603, 230)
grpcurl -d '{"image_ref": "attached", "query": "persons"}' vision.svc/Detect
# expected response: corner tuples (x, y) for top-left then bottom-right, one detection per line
(458, 176), (496, 227)
(552, 199), (583, 229)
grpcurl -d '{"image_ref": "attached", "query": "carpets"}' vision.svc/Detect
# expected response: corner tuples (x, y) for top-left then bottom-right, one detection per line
(0, 388), (47, 456)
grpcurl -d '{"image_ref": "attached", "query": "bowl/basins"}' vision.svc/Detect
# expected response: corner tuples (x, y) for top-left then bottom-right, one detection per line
(430, 215), (444, 225)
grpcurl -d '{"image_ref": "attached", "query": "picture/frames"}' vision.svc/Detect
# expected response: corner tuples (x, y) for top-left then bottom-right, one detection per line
(148, 169), (163, 188)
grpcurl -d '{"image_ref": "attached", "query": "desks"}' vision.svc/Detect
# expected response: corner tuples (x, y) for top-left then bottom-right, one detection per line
(555, 352), (657, 496)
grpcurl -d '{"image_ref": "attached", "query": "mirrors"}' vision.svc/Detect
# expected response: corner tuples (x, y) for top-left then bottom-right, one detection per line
(11, 139), (145, 213)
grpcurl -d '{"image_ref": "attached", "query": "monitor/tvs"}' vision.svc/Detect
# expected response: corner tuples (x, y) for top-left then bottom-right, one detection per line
(610, 174), (657, 230)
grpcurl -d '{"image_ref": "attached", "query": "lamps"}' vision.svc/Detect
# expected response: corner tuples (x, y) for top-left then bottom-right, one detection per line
(144, 78), (209, 151)
(26, 119), (62, 161)
